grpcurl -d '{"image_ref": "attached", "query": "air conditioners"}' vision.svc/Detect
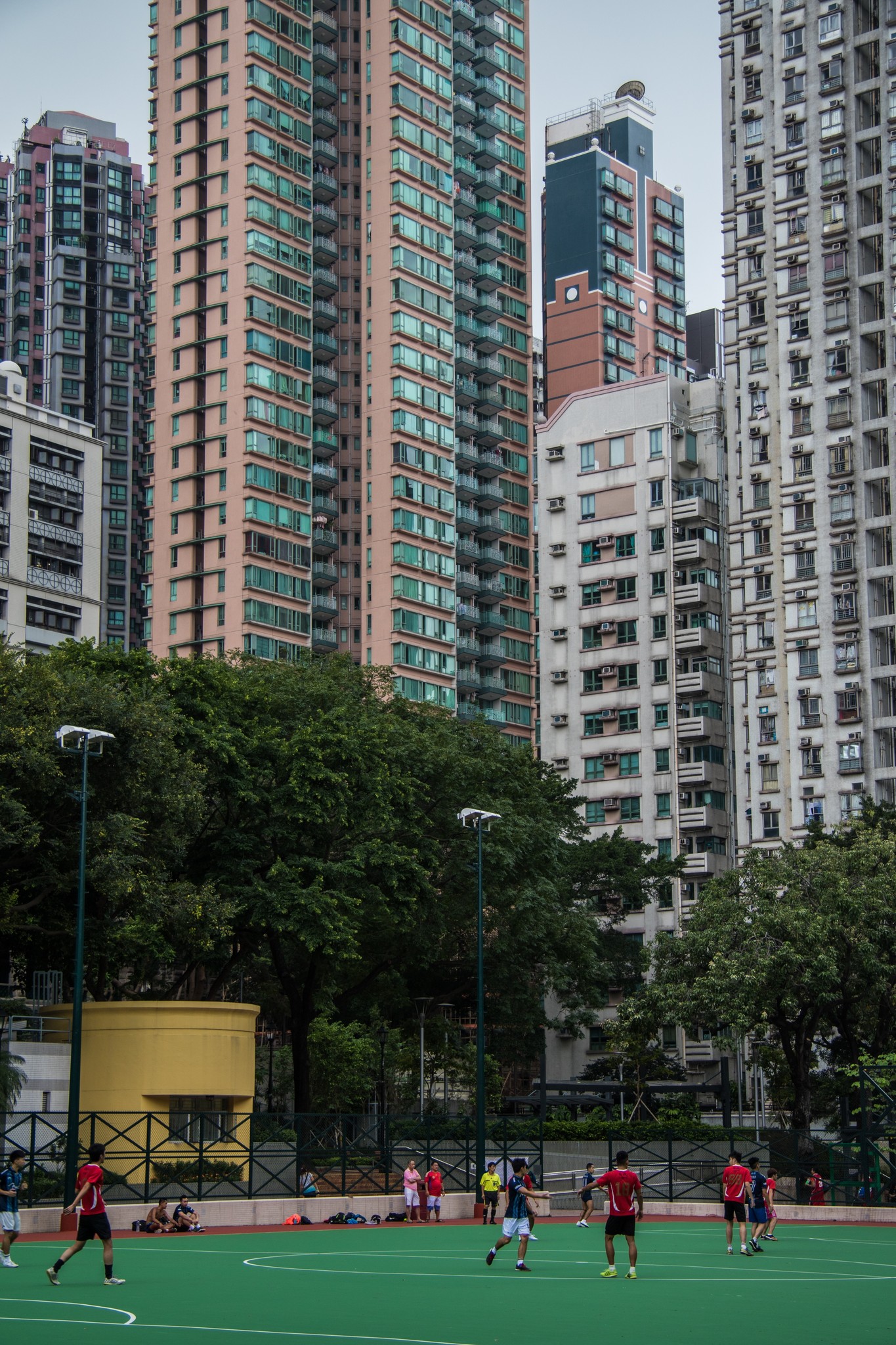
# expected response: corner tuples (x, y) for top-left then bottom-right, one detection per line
(718, 2), (866, 814)
(547, 447), (697, 893)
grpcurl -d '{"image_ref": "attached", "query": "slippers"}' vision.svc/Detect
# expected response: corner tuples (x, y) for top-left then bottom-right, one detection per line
(435, 1219), (445, 1222)
(416, 1220), (426, 1223)
(425, 1219), (430, 1222)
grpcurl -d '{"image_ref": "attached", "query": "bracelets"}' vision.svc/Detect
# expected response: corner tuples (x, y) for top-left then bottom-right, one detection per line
(442, 1189), (445, 1192)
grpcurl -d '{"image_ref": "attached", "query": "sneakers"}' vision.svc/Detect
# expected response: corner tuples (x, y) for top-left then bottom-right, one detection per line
(760, 1233), (778, 1241)
(515, 1262), (531, 1271)
(519, 1235), (538, 1241)
(726, 1249), (733, 1255)
(104, 1277), (126, 1285)
(191, 1227), (206, 1232)
(576, 1219), (589, 1228)
(483, 1220), (497, 1225)
(154, 1228), (177, 1233)
(0, 1257), (19, 1268)
(46, 1267), (61, 1285)
(600, 1269), (618, 1278)
(625, 1271), (637, 1279)
(749, 1239), (764, 1252)
(740, 1247), (754, 1256)
(486, 1248), (496, 1265)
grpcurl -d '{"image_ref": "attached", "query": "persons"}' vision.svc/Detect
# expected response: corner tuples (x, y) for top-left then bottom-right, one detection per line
(145, 1197), (182, 1234)
(852, 1174), (896, 1209)
(805, 1167), (826, 1206)
(479, 1161), (501, 1224)
(485, 1157), (554, 1272)
(575, 1163), (611, 1228)
(422, 1160), (447, 1222)
(171, 1194), (206, 1232)
(743, 1156), (772, 1253)
(573, 1150), (645, 1279)
(0, 1149), (30, 1268)
(722, 1150), (755, 1257)
(403, 1160), (426, 1223)
(760, 1168), (778, 1242)
(294, 1166), (321, 1197)
(505, 1160), (541, 1242)
(46, 1142), (126, 1287)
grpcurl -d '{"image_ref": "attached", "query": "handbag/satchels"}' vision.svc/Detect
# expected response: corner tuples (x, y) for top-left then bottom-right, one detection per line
(282, 1211), (413, 1225)
(132, 1220), (147, 1232)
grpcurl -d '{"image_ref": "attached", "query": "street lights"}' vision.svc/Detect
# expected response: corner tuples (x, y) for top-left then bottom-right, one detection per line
(454, 808), (503, 1213)
(51, 725), (118, 1233)
(615, 1051), (628, 1120)
